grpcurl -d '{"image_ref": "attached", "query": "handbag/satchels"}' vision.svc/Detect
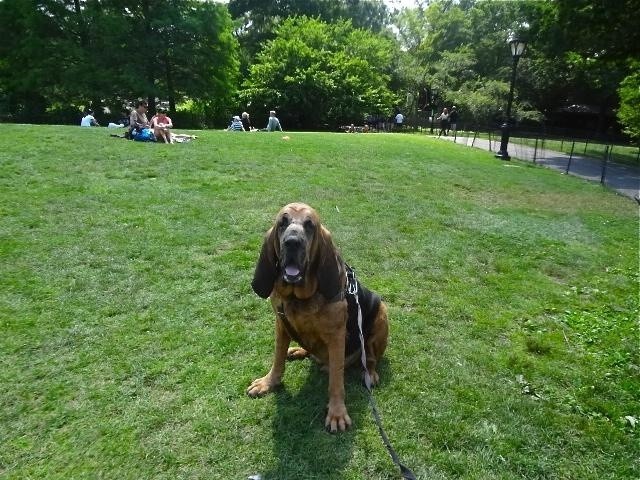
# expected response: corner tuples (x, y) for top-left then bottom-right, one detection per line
(128, 127), (156, 141)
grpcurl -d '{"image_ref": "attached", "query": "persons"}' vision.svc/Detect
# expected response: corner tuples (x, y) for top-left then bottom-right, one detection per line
(362, 125), (370, 133)
(129, 99), (157, 142)
(344, 124), (357, 133)
(224, 116), (246, 131)
(447, 106), (459, 137)
(116, 109), (130, 127)
(394, 111), (404, 132)
(437, 108), (450, 136)
(251, 110), (284, 132)
(152, 107), (175, 145)
(81, 108), (100, 127)
(241, 112), (251, 131)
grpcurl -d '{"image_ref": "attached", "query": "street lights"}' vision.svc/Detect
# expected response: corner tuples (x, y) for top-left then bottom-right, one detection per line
(492, 37), (529, 162)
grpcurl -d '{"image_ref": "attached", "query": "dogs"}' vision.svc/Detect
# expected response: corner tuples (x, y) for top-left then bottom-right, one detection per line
(245, 201), (391, 435)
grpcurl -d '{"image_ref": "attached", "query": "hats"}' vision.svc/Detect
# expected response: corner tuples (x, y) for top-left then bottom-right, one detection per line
(242, 112), (249, 119)
(233, 116), (241, 121)
(157, 108), (167, 113)
(452, 106), (457, 109)
